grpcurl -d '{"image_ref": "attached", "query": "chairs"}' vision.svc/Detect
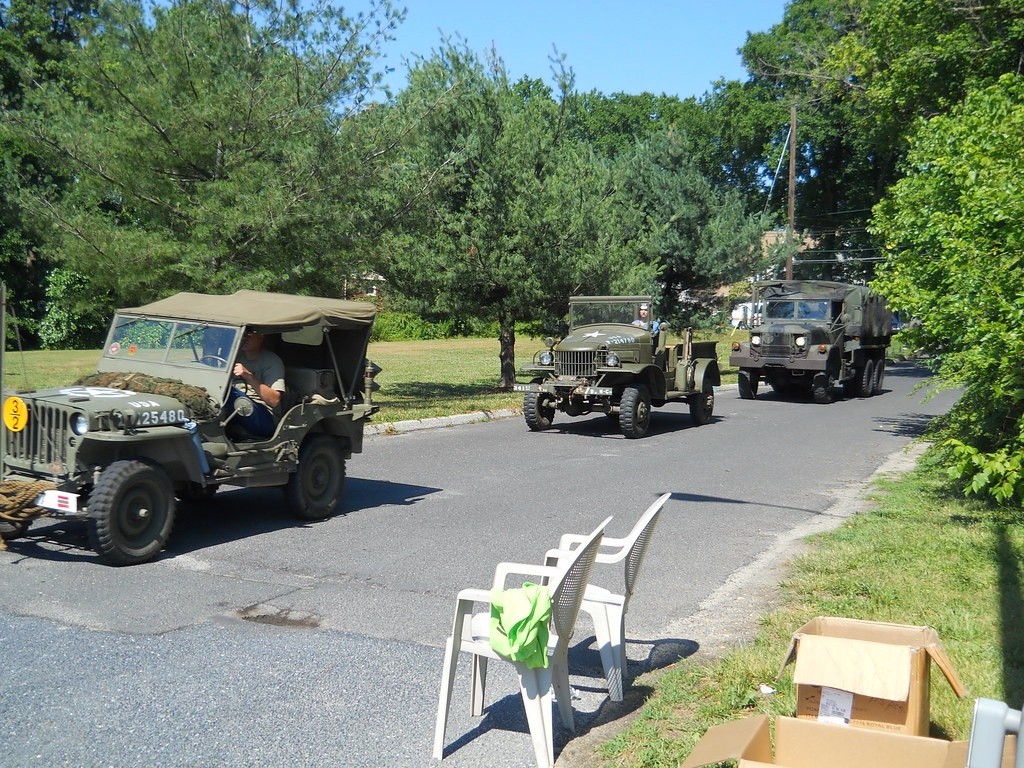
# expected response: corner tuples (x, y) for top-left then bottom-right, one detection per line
(538, 493), (672, 701)
(433, 515), (617, 768)
(653, 331), (667, 369)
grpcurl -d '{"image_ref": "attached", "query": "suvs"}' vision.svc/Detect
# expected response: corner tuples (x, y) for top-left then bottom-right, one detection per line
(1, 284), (381, 566)
(512, 294), (722, 440)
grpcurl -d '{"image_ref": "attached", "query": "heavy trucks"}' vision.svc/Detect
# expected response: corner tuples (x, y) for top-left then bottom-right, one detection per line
(728, 275), (899, 404)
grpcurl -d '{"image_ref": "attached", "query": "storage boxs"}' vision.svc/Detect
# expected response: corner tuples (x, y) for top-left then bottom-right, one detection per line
(778, 616), (967, 736)
(284, 367), (336, 396)
(680, 713), (1019, 768)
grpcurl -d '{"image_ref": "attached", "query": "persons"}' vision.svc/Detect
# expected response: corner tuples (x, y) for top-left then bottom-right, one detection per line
(631, 302), (660, 356)
(216, 326), (286, 437)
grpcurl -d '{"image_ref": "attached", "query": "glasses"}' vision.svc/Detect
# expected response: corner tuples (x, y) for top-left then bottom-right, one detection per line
(639, 309), (647, 311)
(244, 331), (259, 337)
